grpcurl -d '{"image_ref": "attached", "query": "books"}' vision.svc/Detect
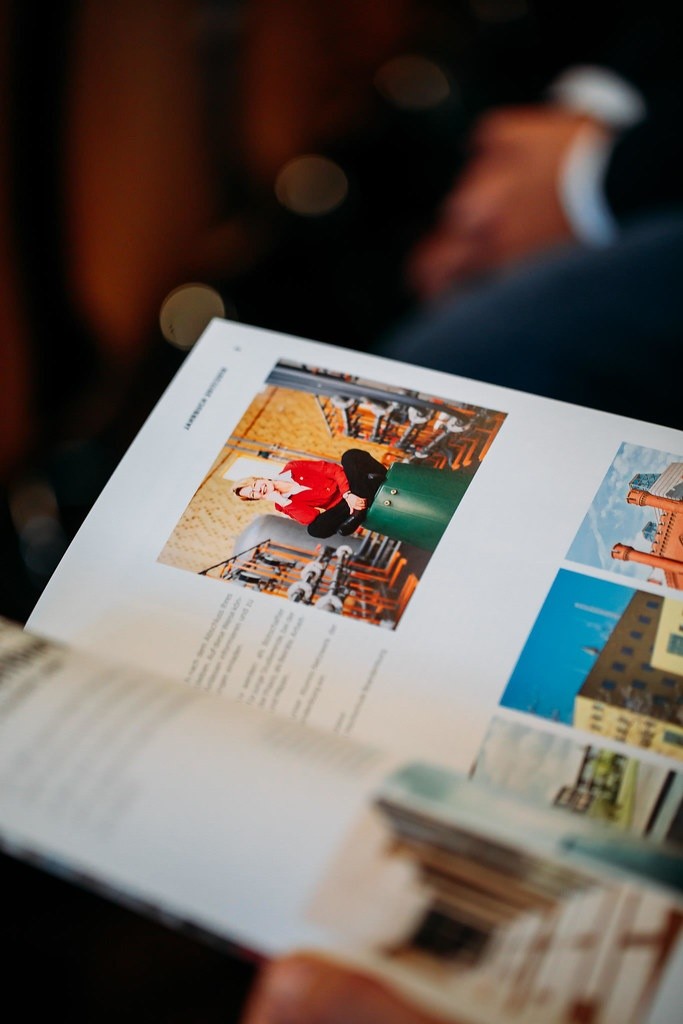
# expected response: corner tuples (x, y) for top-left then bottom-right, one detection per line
(0, 315), (682, 1024)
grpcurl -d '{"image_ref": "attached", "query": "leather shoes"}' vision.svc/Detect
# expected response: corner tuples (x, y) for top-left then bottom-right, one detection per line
(367, 472), (386, 480)
(337, 516), (362, 536)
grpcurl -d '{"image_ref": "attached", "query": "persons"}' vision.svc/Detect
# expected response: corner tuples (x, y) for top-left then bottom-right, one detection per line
(375, 1), (682, 432)
(232, 448), (389, 538)
